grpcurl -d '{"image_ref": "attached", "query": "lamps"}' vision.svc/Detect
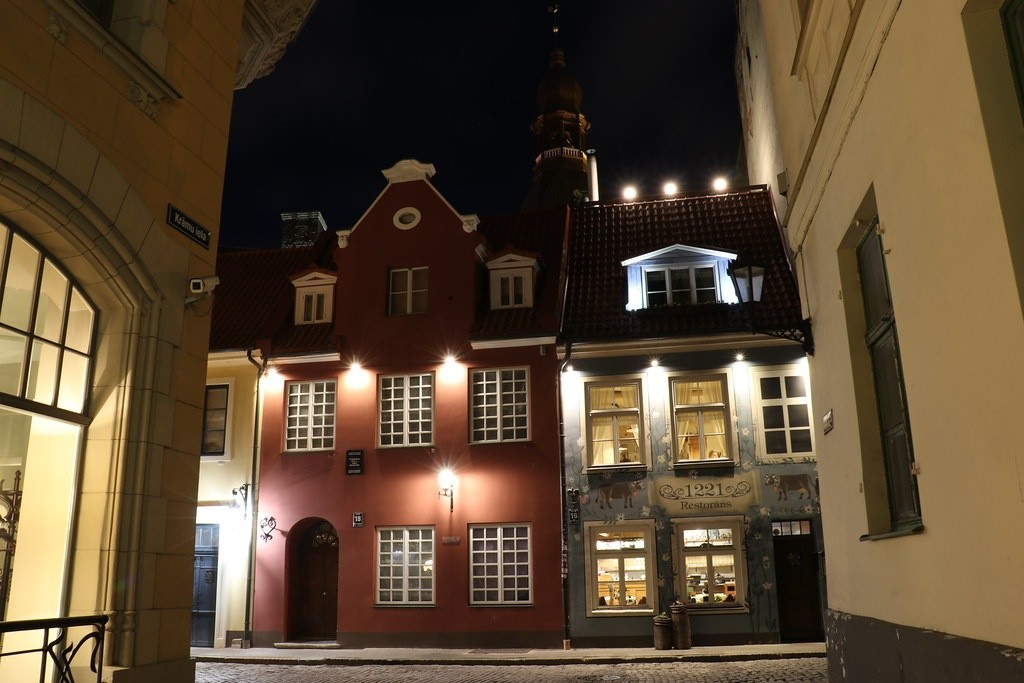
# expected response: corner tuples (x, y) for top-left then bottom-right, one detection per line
(727, 248), (814, 355)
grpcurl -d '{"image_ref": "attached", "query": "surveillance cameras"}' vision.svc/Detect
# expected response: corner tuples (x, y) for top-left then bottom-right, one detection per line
(189, 275), (220, 297)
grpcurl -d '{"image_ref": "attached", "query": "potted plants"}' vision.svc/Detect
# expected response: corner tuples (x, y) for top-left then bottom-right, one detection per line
(669, 590), (689, 613)
(654, 608), (672, 626)
